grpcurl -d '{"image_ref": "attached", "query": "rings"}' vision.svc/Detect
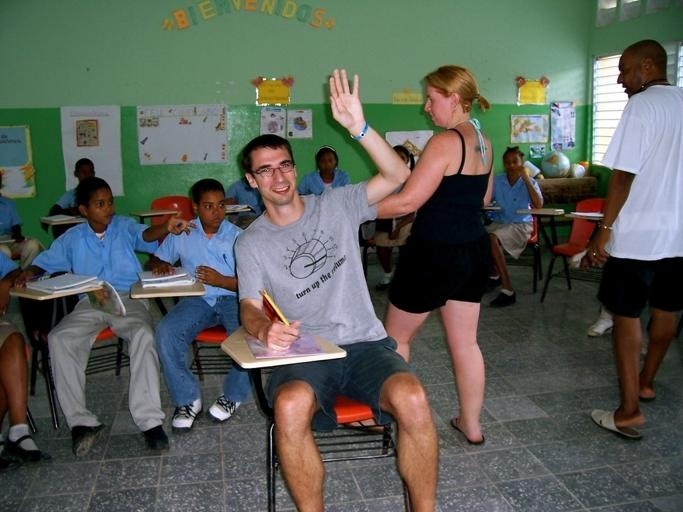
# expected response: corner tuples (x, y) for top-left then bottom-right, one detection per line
(593, 251), (599, 259)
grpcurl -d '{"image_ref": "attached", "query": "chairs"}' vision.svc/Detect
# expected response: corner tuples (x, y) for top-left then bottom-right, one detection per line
(481, 198), (545, 295)
(517, 198), (608, 306)
(363, 239), (375, 280)
(9, 273), (132, 431)
(221, 323), (431, 512)
(129, 197), (195, 244)
(130, 264), (247, 384)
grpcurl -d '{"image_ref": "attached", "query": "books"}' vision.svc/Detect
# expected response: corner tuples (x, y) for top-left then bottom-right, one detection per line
(137, 266), (197, 288)
(25, 272), (127, 316)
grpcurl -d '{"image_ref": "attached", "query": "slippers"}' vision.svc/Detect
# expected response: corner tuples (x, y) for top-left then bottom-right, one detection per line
(637, 390), (656, 405)
(590, 407), (644, 442)
(450, 416), (486, 445)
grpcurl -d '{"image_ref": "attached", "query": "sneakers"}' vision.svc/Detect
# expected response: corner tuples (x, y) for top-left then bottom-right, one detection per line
(69, 422), (106, 458)
(0, 454), (24, 473)
(207, 392), (242, 424)
(171, 396), (203, 435)
(487, 288), (518, 309)
(4, 434), (45, 463)
(143, 424), (170, 453)
(587, 316), (614, 337)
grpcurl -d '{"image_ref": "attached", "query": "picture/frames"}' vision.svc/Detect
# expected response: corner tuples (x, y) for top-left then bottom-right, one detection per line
(255, 76), (293, 107)
(516, 77), (549, 106)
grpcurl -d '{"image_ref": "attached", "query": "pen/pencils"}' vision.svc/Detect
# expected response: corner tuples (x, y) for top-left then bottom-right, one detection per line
(29, 275), (50, 282)
(259, 288), (290, 326)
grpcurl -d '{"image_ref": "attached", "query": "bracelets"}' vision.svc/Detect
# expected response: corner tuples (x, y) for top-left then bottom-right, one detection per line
(596, 221), (613, 232)
(350, 121), (368, 142)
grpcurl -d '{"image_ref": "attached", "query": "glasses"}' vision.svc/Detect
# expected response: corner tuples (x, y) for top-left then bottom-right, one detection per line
(250, 161), (295, 177)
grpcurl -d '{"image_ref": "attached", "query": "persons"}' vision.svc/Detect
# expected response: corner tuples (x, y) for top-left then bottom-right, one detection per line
(375, 65), (500, 446)
(376, 145), (412, 286)
(1, 192), (38, 269)
(42, 157), (96, 238)
(233, 70), (441, 512)
(15, 177), (198, 458)
(226, 175), (262, 218)
(580, 40), (682, 442)
(151, 179), (250, 435)
(0, 251), (52, 474)
(295, 144), (351, 197)
(485, 145), (543, 310)
